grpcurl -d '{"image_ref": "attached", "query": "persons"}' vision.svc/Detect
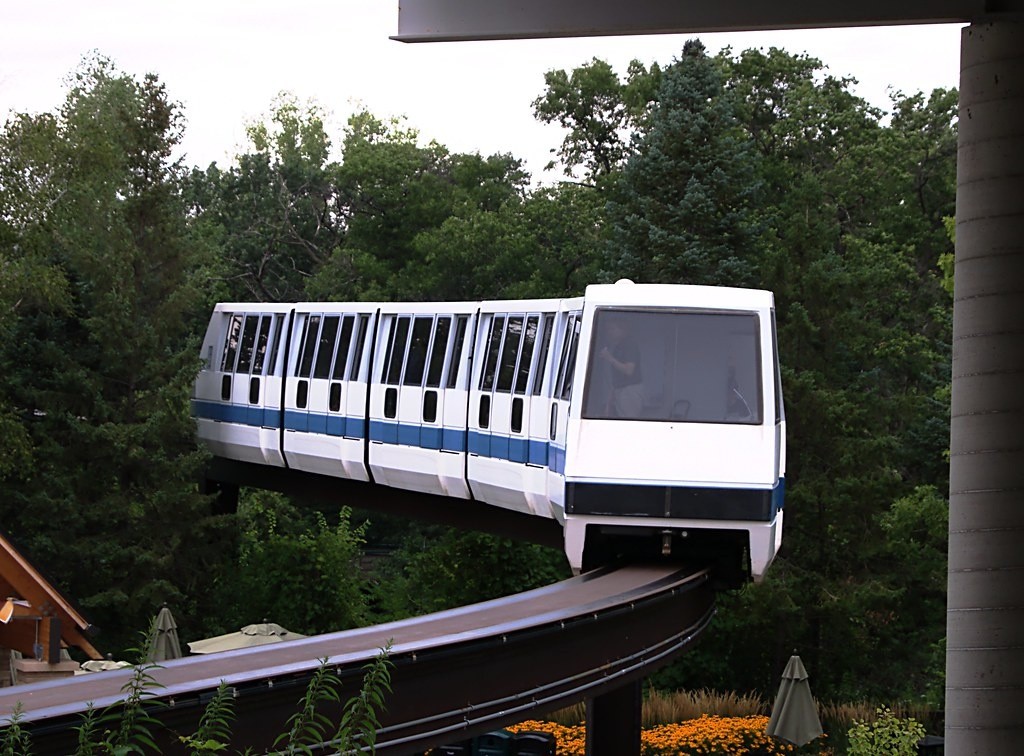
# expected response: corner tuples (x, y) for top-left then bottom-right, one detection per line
(598, 319), (645, 421)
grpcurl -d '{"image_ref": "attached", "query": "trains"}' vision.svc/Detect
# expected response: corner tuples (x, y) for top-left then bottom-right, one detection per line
(190, 278), (789, 588)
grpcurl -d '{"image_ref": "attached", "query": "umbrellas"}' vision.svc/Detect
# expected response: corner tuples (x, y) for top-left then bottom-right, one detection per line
(766, 649), (824, 756)
(78, 601), (317, 707)
